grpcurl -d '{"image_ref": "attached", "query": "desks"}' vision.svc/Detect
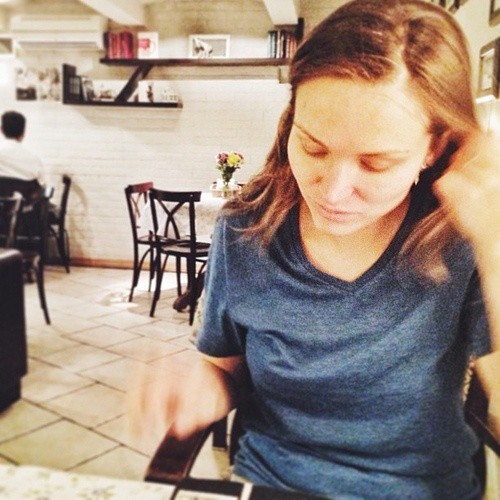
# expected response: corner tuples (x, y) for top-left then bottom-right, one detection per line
(137, 190), (230, 241)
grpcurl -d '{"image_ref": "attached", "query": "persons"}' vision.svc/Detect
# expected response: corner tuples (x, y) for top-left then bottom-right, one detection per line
(121, 0), (500, 500)
(0, 109), (49, 284)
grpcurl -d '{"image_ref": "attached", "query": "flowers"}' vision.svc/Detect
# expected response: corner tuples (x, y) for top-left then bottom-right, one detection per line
(216, 152), (244, 183)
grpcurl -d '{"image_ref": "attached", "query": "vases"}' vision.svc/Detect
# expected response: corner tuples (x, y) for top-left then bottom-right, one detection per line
(221, 186), (232, 199)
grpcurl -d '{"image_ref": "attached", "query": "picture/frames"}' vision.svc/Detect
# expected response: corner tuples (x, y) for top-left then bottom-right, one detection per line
(474, 37), (500, 105)
(431, 0), (500, 27)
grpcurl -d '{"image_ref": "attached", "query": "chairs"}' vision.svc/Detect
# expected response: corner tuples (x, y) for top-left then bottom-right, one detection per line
(143, 409), (500, 481)
(124, 181), (210, 327)
(0, 174), (72, 324)
